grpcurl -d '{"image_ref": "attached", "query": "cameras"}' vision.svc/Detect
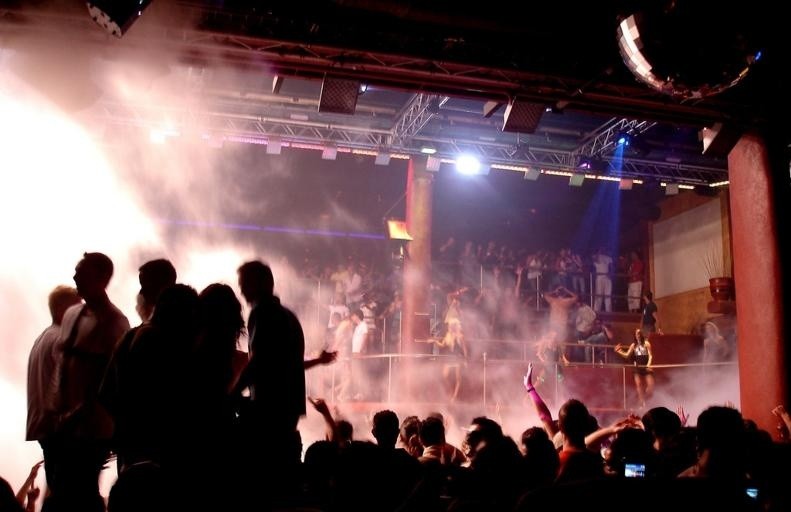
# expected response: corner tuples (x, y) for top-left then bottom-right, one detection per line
(622, 461), (648, 482)
(747, 486), (758, 500)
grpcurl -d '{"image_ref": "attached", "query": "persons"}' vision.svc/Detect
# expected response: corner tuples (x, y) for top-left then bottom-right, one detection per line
(1, 251), (341, 512)
(703, 322), (729, 363)
(304, 362), (790, 511)
(284, 237), (659, 406)
